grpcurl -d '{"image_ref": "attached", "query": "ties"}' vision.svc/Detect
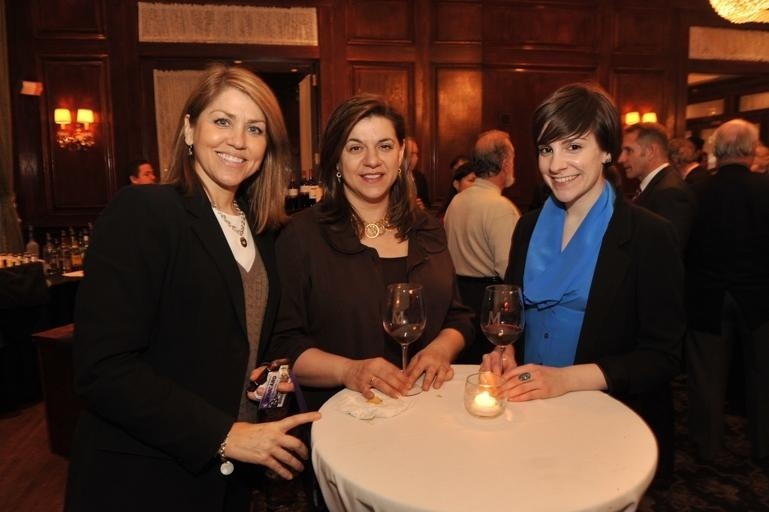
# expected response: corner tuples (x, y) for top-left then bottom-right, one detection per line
(629, 188), (642, 202)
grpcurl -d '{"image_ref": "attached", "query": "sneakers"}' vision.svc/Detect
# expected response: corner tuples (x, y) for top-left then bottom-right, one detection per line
(694, 448), (754, 481)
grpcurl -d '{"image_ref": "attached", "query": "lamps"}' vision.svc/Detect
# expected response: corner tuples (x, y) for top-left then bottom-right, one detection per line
(52, 105), (99, 154)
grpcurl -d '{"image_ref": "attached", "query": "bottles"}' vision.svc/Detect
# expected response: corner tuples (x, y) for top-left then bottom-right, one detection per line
(286, 175), (325, 212)
(26, 225), (91, 276)
(0, 251), (38, 269)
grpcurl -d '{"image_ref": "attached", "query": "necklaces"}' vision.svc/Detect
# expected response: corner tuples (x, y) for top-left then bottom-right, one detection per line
(361, 210), (395, 239)
(213, 200), (247, 249)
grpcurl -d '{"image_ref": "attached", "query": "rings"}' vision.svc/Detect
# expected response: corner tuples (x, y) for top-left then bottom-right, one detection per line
(518, 371), (534, 382)
(368, 374), (376, 388)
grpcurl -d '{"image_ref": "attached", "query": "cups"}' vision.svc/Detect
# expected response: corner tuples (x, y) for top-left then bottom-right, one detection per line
(463, 373), (509, 417)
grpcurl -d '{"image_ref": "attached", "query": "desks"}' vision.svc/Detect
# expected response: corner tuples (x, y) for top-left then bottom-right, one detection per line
(309, 360), (661, 512)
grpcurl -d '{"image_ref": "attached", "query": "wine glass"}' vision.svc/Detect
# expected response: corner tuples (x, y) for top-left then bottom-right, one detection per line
(384, 283), (428, 394)
(479, 283), (526, 374)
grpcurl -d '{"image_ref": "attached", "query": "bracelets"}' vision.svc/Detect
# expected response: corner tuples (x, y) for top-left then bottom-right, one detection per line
(218, 433), (234, 477)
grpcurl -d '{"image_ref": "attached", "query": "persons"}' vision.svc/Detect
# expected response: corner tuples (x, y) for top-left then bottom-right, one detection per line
(441, 129), (525, 365)
(272, 91), (476, 511)
(480, 76), (694, 512)
(61, 63), (322, 512)
(123, 159), (157, 186)
(403, 137), (473, 227)
